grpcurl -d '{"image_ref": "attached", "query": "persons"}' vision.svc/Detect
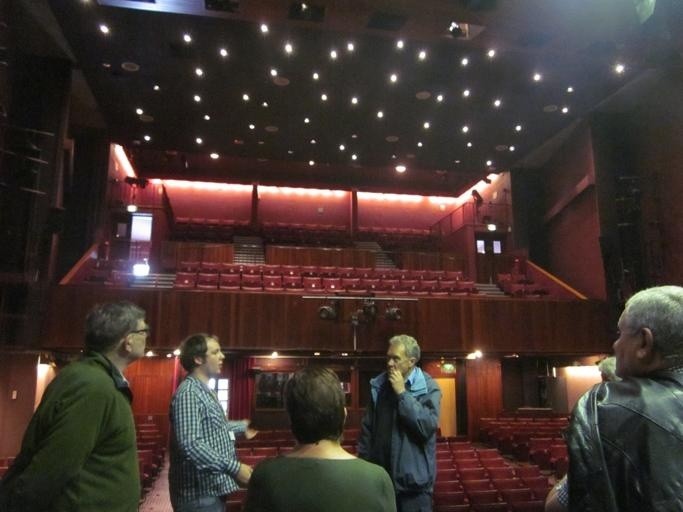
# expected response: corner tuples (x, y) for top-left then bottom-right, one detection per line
(164, 332), (255, 510)
(569, 282), (681, 512)
(357, 335), (440, 509)
(239, 362), (396, 510)
(0, 297), (148, 510)
(540, 357), (623, 510)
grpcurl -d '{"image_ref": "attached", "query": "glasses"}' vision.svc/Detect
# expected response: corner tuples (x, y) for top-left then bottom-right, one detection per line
(130, 326), (152, 336)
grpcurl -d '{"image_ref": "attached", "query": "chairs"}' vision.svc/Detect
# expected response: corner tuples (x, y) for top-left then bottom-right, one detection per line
(134, 420), (167, 489)
(223, 419), (361, 511)
(80, 258), (137, 290)
(432, 408), (573, 512)
(496, 270), (550, 300)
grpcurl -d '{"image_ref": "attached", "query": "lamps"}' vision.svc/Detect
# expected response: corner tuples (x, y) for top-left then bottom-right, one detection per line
(316, 298), (402, 320)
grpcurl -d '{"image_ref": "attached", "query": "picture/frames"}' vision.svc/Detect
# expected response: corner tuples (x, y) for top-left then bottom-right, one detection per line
(251, 368), (291, 413)
